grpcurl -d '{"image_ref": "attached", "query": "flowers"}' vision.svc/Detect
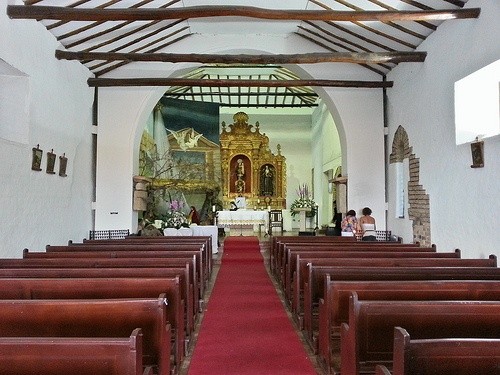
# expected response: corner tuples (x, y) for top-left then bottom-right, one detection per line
(164, 199), (187, 227)
(291, 185), (318, 217)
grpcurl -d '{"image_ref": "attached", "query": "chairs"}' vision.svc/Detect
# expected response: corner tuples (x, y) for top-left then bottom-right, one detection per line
(269, 210), (283, 235)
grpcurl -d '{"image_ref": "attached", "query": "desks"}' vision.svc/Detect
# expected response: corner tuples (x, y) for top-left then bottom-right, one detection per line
(164, 225), (219, 254)
(219, 220), (266, 233)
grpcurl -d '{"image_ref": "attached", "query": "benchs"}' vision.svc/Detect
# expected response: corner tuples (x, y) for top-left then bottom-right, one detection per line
(0, 235), (212, 375)
(270, 236), (500, 375)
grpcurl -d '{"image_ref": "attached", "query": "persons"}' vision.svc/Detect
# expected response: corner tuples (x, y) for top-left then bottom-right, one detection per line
(341, 210), (357, 236)
(189, 206), (200, 225)
(331, 200), (343, 236)
(229, 201), (238, 211)
(360, 207), (376, 240)
(261, 165), (273, 179)
